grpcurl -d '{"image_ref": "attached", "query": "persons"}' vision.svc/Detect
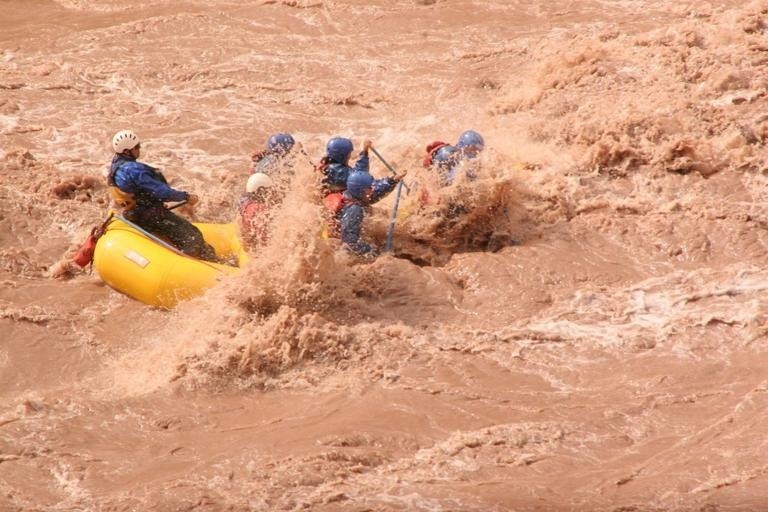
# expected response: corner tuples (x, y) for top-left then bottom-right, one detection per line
(247, 133), (304, 175)
(320, 168), (409, 256)
(315, 135), (375, 196)
(238, 172), (285, 254)
(423, 130), (486, 186)
(107, 129), (222, 264)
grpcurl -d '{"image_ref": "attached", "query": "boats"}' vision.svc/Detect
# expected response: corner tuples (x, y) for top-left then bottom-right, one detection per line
(52, 203), (418, 326)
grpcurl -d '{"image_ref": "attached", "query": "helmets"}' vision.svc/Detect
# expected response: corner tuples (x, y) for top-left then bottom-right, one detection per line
(459, 130), (482, 149)
(326, 137), (353, 161)
(246, 172), (273, 193)
(268, 131), (294, 152)
(347, 170), (374, 198)
(112, 129), (140, 153)
